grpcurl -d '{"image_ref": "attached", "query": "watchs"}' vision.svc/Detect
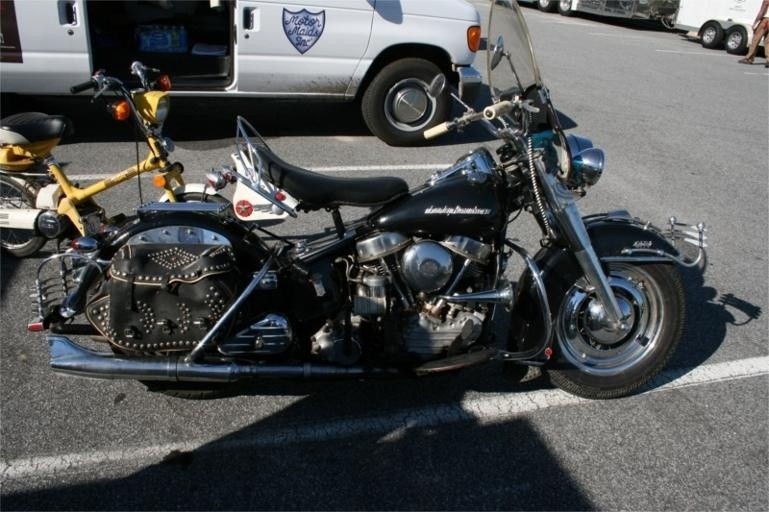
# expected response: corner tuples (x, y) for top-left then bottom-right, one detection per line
(28, 0), (708, 399)
(1, 57), (235, 257)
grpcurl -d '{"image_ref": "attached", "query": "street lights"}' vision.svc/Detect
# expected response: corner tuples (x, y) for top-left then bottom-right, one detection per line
(86, 244), (238, 350)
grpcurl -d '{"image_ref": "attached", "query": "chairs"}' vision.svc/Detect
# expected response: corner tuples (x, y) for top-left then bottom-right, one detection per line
(739, 58), (754, 64)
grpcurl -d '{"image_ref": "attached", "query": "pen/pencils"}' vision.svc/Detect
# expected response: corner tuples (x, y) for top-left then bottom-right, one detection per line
(0, 0), (486, 149)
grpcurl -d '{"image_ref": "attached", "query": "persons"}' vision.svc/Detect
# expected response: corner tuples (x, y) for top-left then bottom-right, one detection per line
(738, 0), (769, 71)
